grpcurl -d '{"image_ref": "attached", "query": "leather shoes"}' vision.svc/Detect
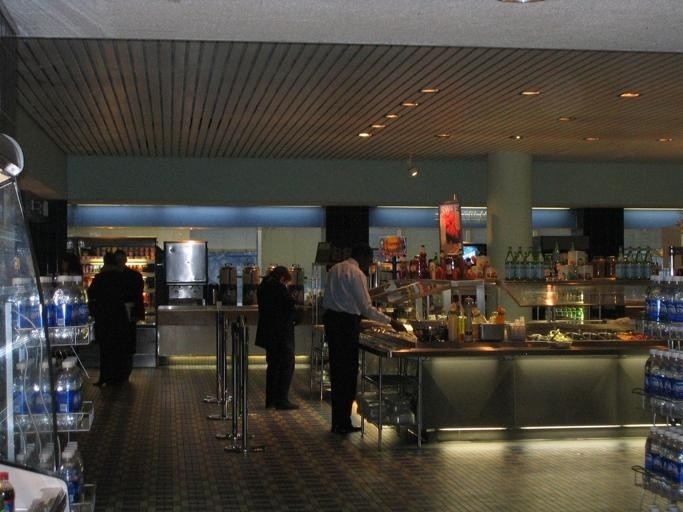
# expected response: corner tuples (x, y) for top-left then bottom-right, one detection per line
(275, 401), (299, 409)
(332, 424), (362, 433)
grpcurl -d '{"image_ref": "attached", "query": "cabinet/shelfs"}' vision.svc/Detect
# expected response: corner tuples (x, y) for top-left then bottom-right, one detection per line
(72, 238), (163, 368)
(310, 327), (332, 400)
(361, 345), (422, 451)
(632, 319), (683, 512)
(6, 303), (95, 512)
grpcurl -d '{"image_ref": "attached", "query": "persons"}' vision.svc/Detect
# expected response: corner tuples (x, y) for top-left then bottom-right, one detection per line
(115, 249), (145, 358)
(322, 246), (408, 434)
(88, 252), (133, 386)
(257, 266), (310, 409)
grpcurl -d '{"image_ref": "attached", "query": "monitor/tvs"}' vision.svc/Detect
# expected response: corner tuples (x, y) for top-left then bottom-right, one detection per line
(461, 242), (488, 267)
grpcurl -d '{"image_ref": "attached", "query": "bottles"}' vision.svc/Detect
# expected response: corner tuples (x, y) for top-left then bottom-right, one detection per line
(1, 274), (89, 508)
(553, 306), (584, 324)
(644, 274), (683, 495)
(503, 244), (659, 281)
(378, 245), (492, 282)
(448, 296), (474, 345)
